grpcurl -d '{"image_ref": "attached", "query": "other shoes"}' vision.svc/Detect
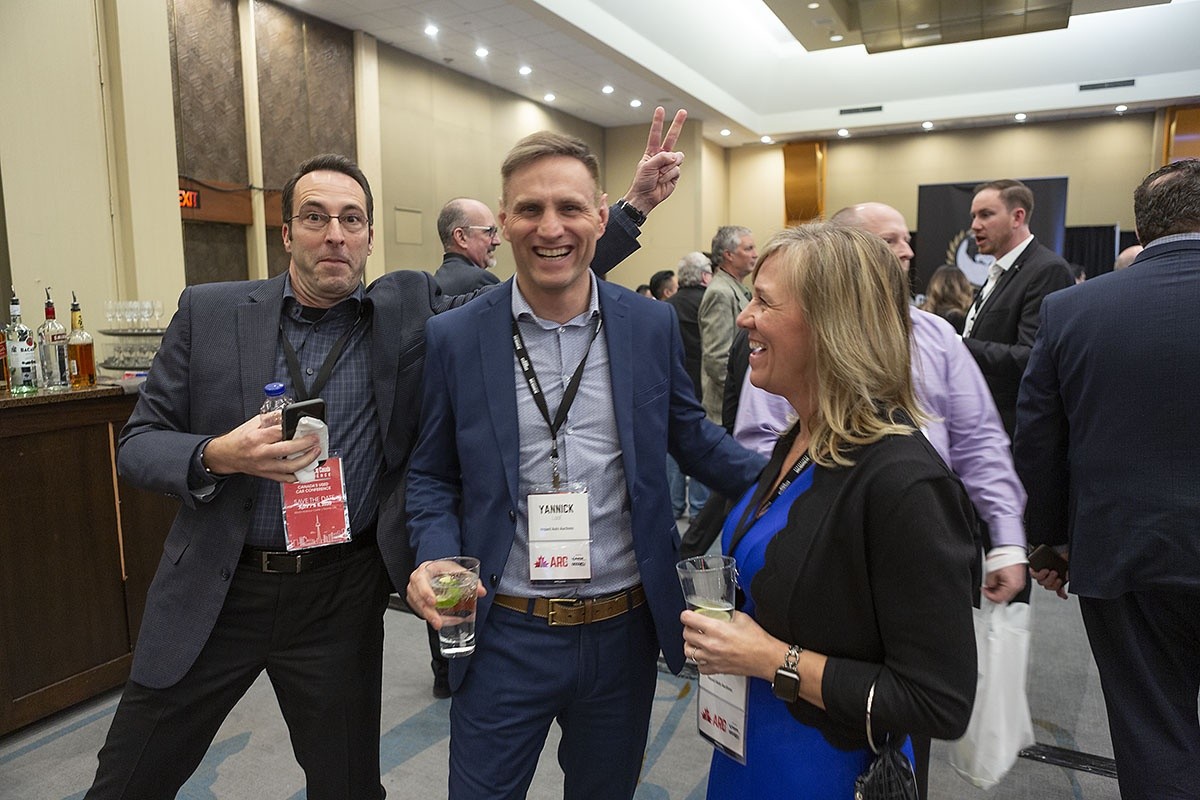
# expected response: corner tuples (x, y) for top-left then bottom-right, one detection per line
(433, 687), (450, 699)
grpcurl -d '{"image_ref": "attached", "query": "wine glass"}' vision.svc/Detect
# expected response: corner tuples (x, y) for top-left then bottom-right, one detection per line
(101, 298), (166, 368)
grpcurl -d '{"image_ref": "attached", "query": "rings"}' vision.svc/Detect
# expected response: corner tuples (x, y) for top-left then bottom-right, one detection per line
(690, 647), (696, 663)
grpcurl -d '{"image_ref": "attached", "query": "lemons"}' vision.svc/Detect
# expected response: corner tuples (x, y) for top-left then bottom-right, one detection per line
(434, 579), (460, 609)
(694, 609), (730, 622)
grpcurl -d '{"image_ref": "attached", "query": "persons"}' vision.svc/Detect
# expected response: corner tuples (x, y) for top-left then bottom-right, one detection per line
(636, 179), (1144, 800)
(428, 198), (502, 699)
(405, 131), (772, 800)
(1012, 161), (1200, 800)
(85, 106), (687, 800)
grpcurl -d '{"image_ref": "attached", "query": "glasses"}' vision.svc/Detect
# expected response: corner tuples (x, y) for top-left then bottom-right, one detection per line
(285, 211), (372, 228)
(452, 225), (498, 238)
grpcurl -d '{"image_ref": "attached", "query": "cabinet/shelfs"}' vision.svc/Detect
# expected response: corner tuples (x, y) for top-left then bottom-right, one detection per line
(0, 396), (187, 738)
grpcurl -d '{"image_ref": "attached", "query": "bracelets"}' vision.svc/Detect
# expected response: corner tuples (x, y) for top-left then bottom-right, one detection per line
(616, 199), (647, 228)
(200, 453), (213, 475)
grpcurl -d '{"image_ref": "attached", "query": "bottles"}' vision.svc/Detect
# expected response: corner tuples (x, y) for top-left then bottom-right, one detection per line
(258, 382), (297, 464)
(0, 324), (9, 390)
(6, 284), (38, 395)
(34, 289), (69, 392)
(64, 290), (99, 390)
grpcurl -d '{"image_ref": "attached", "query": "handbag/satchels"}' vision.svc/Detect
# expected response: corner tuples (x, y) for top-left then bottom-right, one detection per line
(948, 596), (1036, 791)
(854, 678), (919, 800)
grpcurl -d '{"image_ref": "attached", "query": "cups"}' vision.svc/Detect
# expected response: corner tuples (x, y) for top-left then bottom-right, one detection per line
(427, 556), (480, 658)
(675, 555), (736, 633)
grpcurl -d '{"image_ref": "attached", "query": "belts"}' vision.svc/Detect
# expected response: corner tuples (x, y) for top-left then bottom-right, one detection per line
(492, 586), (646, 626)
(238, 540), (365, 573)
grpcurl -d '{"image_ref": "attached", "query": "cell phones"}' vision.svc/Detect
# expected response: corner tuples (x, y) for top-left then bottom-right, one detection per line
(282, 399), (326, 467)
(1027, 542), (1069, 588)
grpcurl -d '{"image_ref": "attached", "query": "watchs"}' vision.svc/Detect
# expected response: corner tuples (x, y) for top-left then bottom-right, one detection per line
(770, 645), (802, 705)
